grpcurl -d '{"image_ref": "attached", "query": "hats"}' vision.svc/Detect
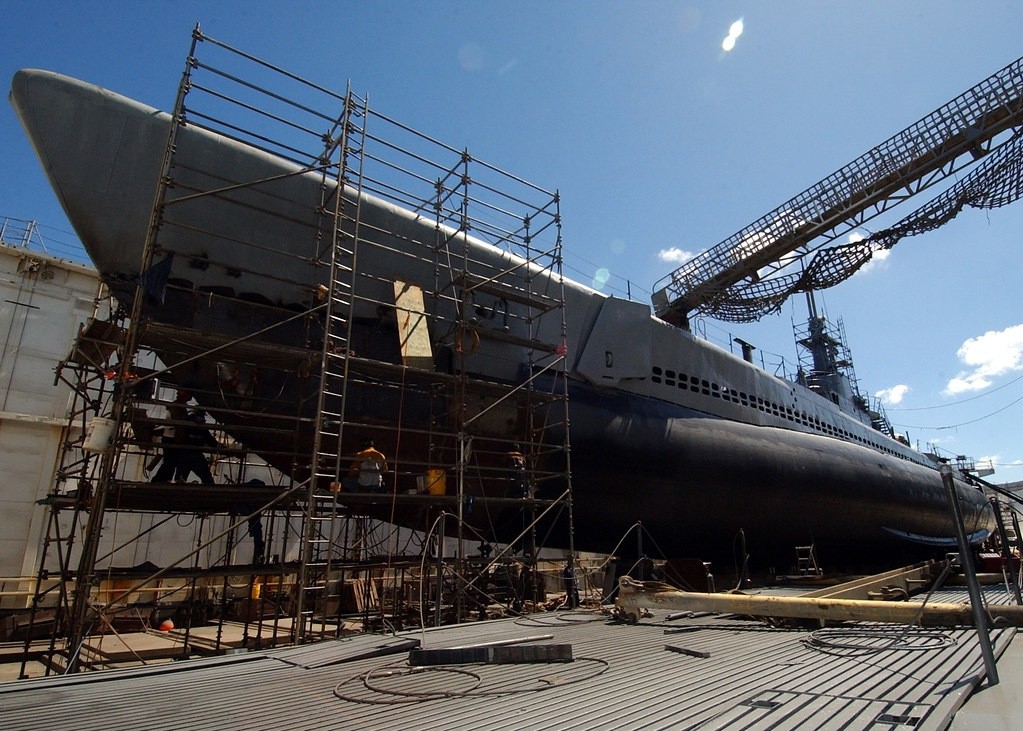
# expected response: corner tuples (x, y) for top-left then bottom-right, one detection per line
(195, 405), (207, 415)
(513, 443), (519, 449)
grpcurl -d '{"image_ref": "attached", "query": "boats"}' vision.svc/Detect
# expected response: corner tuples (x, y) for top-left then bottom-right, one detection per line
(9, 63), (999, 604)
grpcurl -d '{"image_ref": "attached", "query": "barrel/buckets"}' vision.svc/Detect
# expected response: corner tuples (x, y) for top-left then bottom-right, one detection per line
(82, 417), (117, 453)
(425, 468), (447, 495)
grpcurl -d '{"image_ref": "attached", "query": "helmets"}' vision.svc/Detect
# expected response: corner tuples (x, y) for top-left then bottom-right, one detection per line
(177, 388), (192, 400)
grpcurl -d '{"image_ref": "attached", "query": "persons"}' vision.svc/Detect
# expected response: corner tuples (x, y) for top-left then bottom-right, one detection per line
(344, 438), (388, 494)
(151, 387), (246, 484)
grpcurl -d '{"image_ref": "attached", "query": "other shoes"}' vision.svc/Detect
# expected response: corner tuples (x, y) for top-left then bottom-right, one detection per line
(151, 478), (166, 484)
(175, 477), (185, 483)
